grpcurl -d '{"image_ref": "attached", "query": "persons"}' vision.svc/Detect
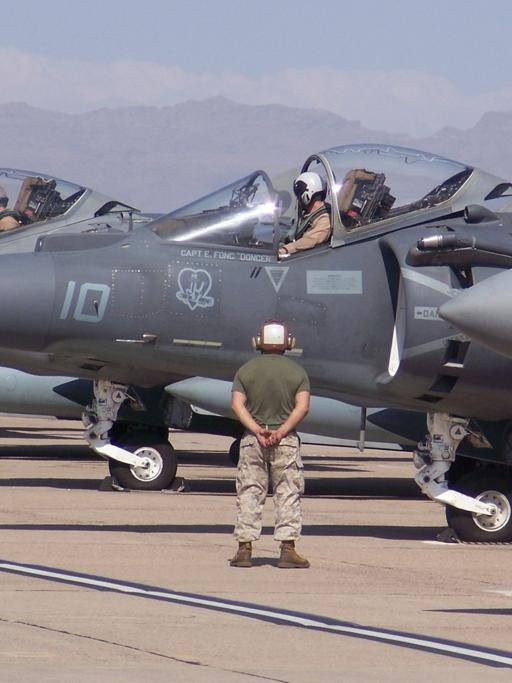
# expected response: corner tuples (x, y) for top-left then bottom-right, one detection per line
(278, 171), (332, 256)
(230, 318), (310, 569)
(0, 185), (21, 231)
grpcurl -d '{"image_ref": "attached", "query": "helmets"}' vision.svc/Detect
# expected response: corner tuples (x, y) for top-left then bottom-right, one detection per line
(294, 170), (328, 206)
(252, 319), (297, 352)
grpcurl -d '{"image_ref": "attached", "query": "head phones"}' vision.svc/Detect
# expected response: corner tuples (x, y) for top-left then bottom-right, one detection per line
(251, 332), (297, 352)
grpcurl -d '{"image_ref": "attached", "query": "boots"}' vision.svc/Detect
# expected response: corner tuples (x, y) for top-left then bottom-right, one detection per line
(278, 541), (309, 568)
(230, 542), (252, 567)
